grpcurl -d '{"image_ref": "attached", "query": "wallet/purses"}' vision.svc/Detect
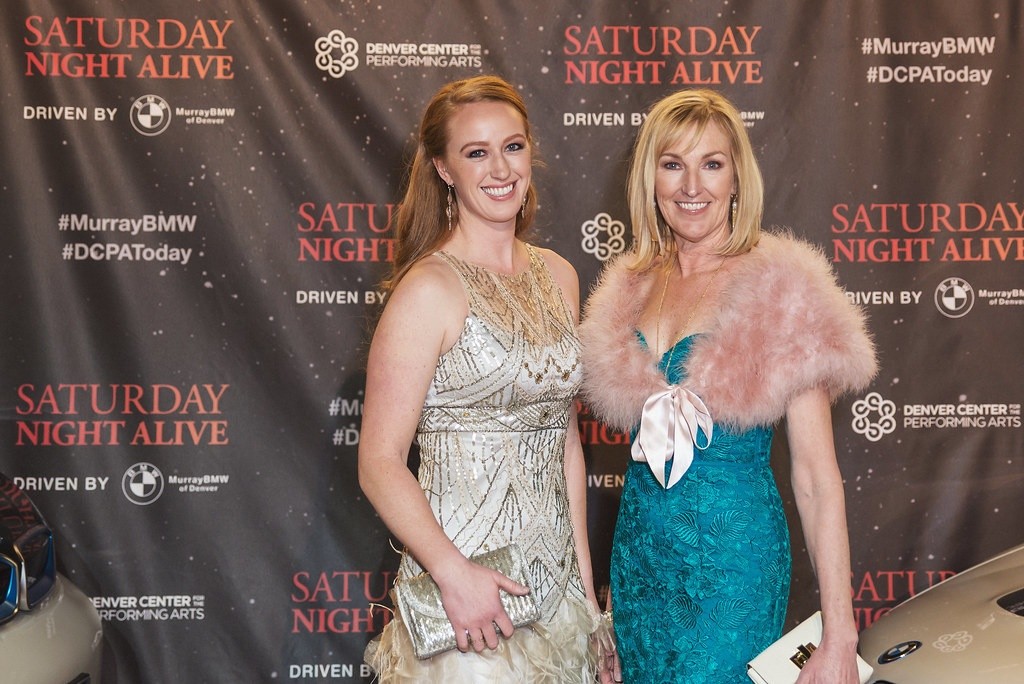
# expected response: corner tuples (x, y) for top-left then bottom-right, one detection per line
(394, 545), (542, 660)
(746, 610), (874, 684)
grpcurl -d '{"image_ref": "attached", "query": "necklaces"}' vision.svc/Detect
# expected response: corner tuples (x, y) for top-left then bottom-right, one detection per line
(654, 250), (727, 381)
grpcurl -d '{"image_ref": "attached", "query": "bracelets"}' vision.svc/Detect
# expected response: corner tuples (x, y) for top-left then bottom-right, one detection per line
(599, 610), (615, 623)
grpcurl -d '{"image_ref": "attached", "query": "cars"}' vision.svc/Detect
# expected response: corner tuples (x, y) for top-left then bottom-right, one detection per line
(849, 542), (1024, 684)
(0, 463), (105, 684)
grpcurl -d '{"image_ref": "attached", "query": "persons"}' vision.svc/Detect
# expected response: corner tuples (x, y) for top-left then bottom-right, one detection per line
(578, 88), (879, 684)
(357, 77), (617, 684)
